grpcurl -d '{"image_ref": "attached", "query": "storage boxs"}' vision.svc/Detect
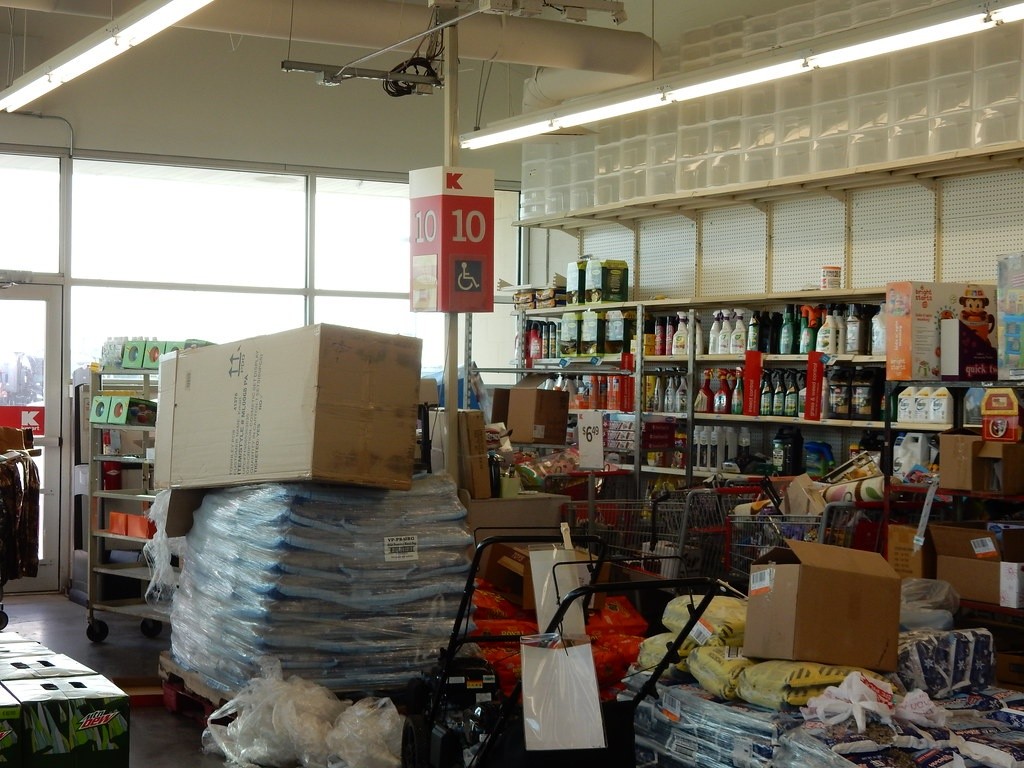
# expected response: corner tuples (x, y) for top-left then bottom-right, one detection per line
(154, 324), (421, 491)
(930, 520), (1023, 608)
(889, 524), (936, 579)
(0, 632), (131, 762)
(741, 539), (899, 673)
(779, 473), (827, 531)
(886, 281), (998, 381)
(938, 427), (989, 491)
(428, 374), (600, 614)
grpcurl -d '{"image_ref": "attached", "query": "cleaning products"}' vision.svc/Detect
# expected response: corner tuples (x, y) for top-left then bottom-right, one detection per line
(827, 364), (954, 425)
(746, 300), (887, 356)
(771, 425), (804, 477)
(654, 307), (746, 356)
(692, 424), (751, 473)
(523, 319), (562, 360)
(651, 366), (807, 418)
(544, 372), (635, 413)
(892, 431), (930, 484)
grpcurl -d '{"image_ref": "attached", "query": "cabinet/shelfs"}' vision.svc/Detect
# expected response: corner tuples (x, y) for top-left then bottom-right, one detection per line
(86, 368), (172, 643)
(518, 287), (982, 486)
